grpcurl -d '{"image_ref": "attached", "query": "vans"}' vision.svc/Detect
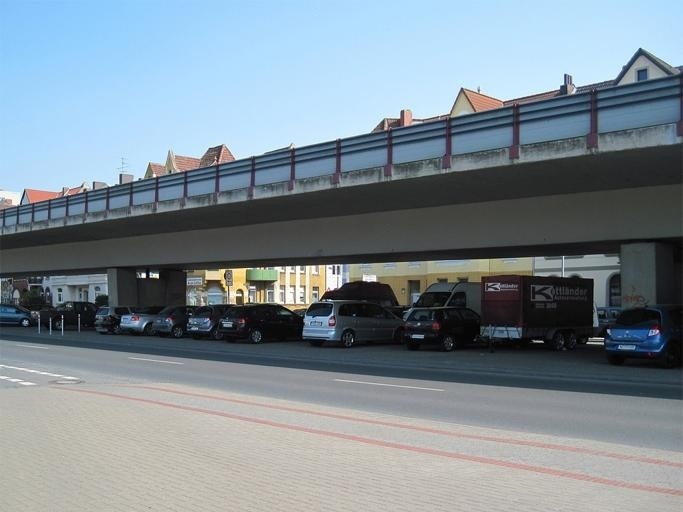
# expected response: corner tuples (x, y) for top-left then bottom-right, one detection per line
(320, 282), (481, 314)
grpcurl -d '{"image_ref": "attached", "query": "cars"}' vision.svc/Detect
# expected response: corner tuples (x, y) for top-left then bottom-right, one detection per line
(597, 307), (683, 368)
